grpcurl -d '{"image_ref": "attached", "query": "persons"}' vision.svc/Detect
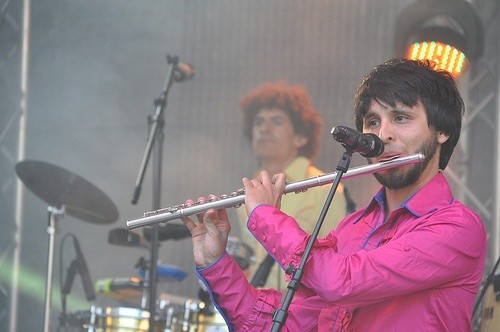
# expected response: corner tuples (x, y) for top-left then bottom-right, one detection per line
(177, 56), (486, 332)
(228, 81), (349, 294)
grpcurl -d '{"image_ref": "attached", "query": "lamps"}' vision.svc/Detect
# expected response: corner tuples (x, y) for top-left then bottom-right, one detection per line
(405, 28), (472, 78)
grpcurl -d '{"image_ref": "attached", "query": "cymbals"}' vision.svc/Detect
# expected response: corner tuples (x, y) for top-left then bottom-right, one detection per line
(14, 160), (119, 225)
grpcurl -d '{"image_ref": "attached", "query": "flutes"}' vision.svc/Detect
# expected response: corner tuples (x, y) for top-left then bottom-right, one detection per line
(126, 152), (426, 230)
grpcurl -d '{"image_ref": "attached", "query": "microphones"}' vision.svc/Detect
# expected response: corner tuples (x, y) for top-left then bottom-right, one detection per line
(168, 56), (194, 82)
(331, 126), (384, 158)
(108, 229), (152, 248)
(73, 236), (96, 301)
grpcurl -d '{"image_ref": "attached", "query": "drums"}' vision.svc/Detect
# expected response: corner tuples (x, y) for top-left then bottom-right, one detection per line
(87, 305), (151, 332)
(225, 237), (254, 269)
(153, 298), (229, 332)
